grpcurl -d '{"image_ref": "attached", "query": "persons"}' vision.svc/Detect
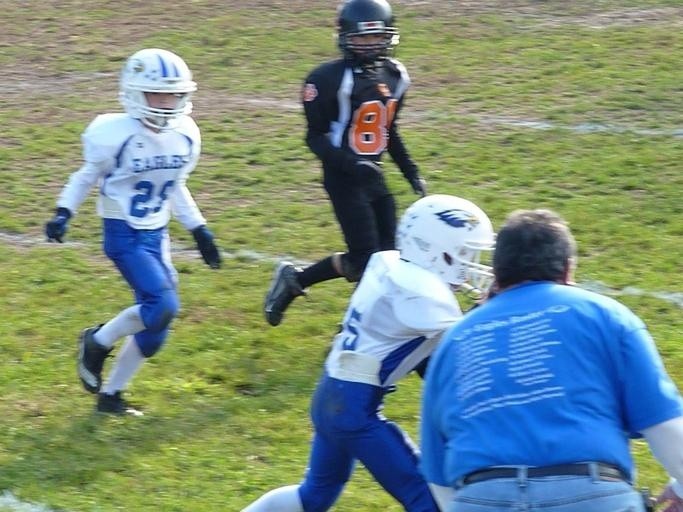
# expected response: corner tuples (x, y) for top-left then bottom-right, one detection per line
(261, 2), (429, 326)
(233, 192), (497, 512)
(415, 207), (682, 512)
(44, 47), (221, 420)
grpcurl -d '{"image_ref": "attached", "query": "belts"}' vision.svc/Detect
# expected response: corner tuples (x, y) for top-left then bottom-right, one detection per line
(455, 461), (630, 484)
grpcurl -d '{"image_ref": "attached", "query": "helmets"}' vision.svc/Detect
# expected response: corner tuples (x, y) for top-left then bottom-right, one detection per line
(335, 0), (397, 60)
(394, 193), (496, 292)
(118, 44), (197, 125)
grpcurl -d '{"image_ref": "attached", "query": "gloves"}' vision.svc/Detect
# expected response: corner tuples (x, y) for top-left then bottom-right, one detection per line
(355, 155), (384, 179)
(43, 207), (74, 243)
(412, 178), (430, 198)
(194, 227), (220, 270)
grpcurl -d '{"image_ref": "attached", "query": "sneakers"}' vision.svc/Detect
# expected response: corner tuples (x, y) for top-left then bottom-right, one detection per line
(78, 322), (111, 394)
(98, 390), (144, 418)
(264, 260), (307, 329)
